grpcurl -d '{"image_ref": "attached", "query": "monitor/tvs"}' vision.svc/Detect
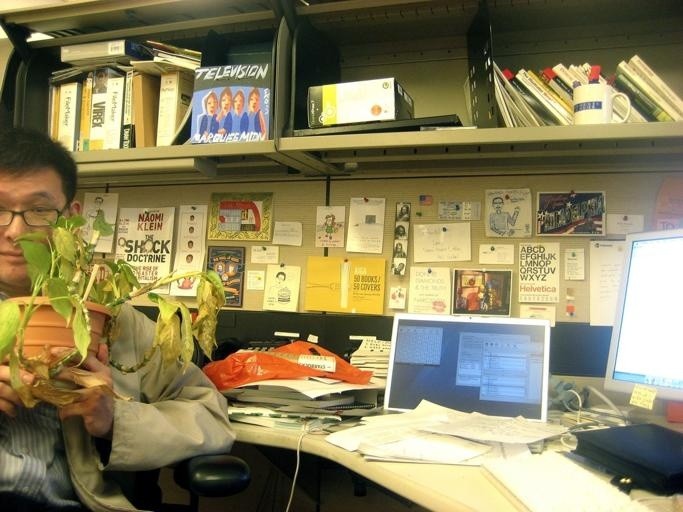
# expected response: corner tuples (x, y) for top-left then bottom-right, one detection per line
(604, 227), (683, 403)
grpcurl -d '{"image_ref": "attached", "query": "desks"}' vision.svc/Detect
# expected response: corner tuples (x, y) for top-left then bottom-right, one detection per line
(227, 400), (683, 512)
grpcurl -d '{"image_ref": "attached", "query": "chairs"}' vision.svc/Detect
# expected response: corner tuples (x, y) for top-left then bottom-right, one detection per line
(97, 433), (252, 512)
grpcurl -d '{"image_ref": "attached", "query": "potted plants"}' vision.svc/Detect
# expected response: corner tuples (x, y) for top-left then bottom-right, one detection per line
(0, 208), (226, 410)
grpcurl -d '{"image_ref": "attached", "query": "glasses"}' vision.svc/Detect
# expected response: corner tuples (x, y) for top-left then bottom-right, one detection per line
(0, 201), (70, 228)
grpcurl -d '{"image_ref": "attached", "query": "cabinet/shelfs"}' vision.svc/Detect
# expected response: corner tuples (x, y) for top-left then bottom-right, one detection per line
(1, 0), (683, 186)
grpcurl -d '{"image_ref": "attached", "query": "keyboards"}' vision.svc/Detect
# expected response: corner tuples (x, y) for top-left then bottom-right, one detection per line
(482, 449), (651, 512)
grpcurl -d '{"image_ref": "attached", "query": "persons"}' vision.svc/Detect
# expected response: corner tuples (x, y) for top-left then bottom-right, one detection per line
(394, 243), (405, 258)
(491, 196), (518, 235)
(395, 225), (408, 239)
(394, 262), (404, 274)
(397, 205), (410, 221)
(0, 125), (236, 511)
(195, 87), (266, 142)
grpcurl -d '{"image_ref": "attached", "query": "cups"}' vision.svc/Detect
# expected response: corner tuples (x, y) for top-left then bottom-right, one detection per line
(570, 83), (632, 124)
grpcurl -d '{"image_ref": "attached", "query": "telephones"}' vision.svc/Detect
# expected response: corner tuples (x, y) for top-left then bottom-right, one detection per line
(239, 338), (291, 352)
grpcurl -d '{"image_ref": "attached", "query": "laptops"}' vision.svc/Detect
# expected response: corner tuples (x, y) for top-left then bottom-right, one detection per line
(383, 312), (551, 453)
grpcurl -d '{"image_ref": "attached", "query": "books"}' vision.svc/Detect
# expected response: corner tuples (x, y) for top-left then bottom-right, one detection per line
(45, 42), (201, 148)
(490, 51), (681, 129)
(216, 375), (386, 430)
